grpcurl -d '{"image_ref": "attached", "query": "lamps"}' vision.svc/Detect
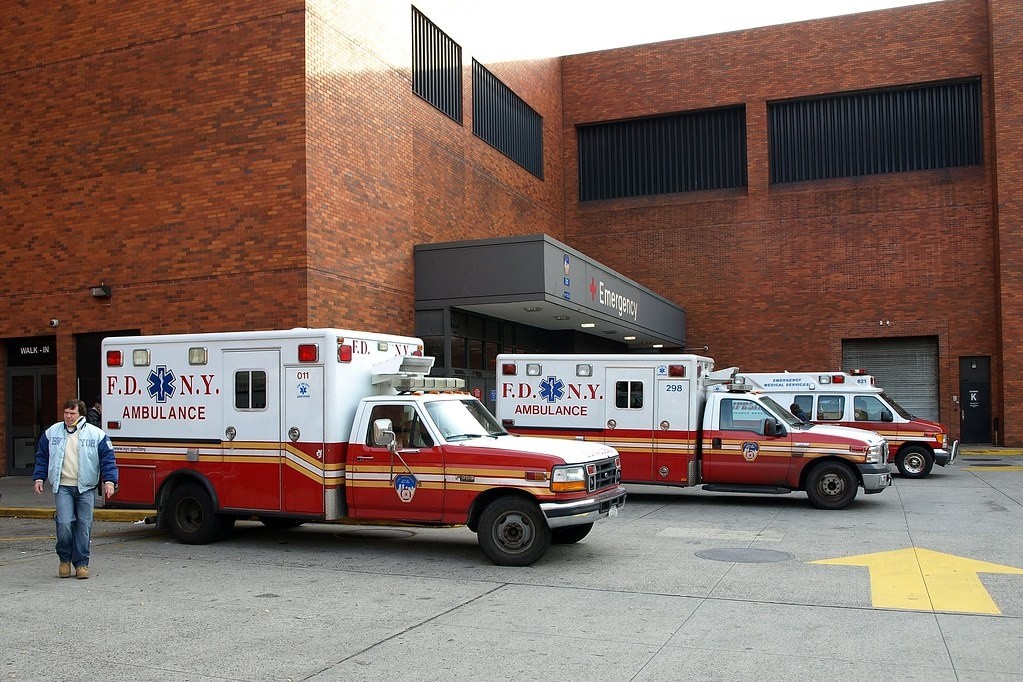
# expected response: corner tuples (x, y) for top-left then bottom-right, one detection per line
(88, 285), (112, 297)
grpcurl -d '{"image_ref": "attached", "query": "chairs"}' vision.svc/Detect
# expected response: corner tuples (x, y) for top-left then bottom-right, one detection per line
(401, 420), (425, 447)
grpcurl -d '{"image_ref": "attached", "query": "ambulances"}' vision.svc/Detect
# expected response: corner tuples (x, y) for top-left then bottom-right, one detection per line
(734, 368), (959, 480)
(97, 323), (630, 568)
(494, 352), (893, 512)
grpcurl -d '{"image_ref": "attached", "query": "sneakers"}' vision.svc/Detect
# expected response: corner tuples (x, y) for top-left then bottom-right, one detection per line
(59, 561), (71, 577)
(75, 565), (89, 579)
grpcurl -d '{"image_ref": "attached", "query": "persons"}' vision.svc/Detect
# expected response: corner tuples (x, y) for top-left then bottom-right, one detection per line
(790, 403), (808, 423)
(33, 399), (119, 580)
(86, 402), (103, 429)
